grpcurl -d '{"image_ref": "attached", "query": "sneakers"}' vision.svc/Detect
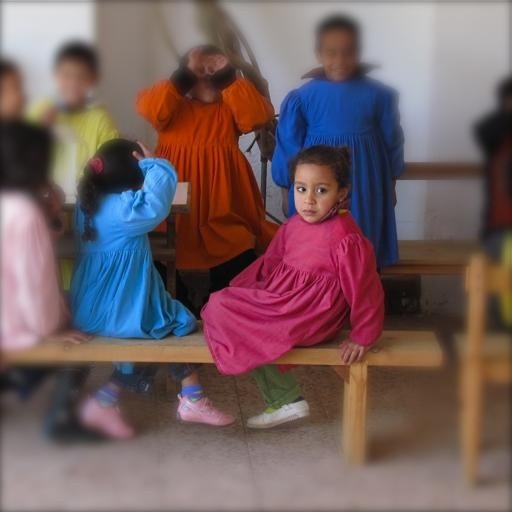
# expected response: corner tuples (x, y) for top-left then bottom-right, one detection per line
(246, 397), (309, 429)
(75, 395), (134, 439)
(175, 394), (237, 430)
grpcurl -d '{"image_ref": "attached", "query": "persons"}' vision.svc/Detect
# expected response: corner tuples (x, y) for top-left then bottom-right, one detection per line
(68, 137), (235, 445)
(0, 59), (26, 122)
(473, 74), (511, 335)
(133, 45), (275, 321)
(0, 120), (93, 445)
(26, 41), (118, 295)
(198, 145), (386, 430)
(272, 14), (405, 274)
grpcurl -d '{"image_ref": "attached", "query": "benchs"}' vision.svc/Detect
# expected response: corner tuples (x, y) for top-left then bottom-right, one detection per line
(372, 237), (481, 279)
(0, 320), (443, 467)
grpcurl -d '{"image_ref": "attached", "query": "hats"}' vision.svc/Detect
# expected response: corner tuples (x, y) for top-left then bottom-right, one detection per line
(161, 1), (230, 60)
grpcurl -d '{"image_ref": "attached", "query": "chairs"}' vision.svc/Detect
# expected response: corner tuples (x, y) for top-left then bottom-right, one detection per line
(447, 251), (512, 495)
